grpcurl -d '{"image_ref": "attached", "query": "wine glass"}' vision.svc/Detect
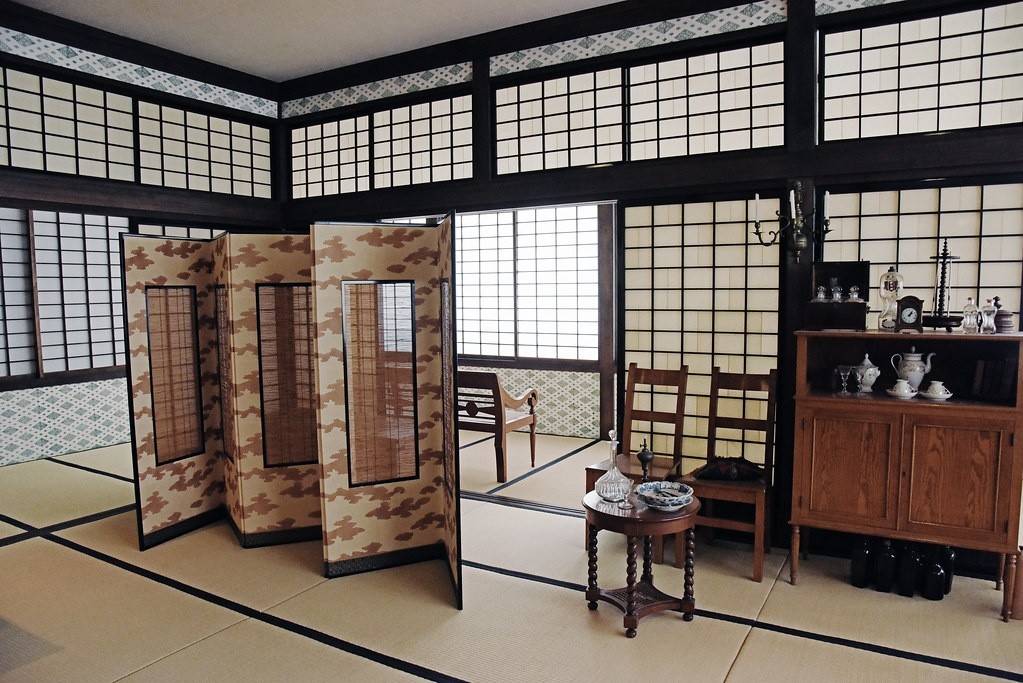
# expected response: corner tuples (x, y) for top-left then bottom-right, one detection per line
(837, 365), (853, 395)
(618, 478), (634, 509)
(853, 366), (869, 396)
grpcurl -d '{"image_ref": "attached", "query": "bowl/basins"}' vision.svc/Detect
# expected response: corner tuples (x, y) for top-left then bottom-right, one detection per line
(636, 481), (694, 504)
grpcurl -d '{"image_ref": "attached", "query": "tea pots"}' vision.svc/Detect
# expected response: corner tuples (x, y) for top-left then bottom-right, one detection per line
(890, 345), (936, 393)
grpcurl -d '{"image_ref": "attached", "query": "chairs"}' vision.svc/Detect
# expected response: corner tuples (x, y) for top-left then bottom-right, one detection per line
(586, 362), (690, 563)
(455, 368), (539, 483)
(674, 367), (780, 584)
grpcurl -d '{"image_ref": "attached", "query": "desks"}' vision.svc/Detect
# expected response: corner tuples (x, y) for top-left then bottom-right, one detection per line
(582, 483), (702, 639)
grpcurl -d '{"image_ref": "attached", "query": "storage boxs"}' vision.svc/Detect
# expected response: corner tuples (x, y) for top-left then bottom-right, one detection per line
(804, 261), (870, 331)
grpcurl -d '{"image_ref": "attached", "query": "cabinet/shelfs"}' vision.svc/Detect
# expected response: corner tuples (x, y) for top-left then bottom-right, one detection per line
(790, 328), (1023, 622)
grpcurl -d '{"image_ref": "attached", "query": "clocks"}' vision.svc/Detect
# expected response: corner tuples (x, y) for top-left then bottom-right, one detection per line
(894, 295), (924, 333)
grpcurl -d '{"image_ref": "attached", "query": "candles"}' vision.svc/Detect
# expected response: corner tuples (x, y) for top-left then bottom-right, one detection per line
(790, 189), (796, 219)
(825, 190), (829, 220)
(755, 193), (759, 222)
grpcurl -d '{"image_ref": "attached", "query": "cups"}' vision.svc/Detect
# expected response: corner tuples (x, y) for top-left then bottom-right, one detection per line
(894, 378), (911, 393)
(927, 381), (946, 395)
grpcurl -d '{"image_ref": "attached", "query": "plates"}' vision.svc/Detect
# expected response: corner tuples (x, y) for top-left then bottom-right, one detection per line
(637, 495), (694, 511)
(885, 389), (918, 399)
(920, 391), (954, 401)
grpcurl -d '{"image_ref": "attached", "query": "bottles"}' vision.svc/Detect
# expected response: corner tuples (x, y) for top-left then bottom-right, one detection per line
(980, 298), (997, 334)
(595, 430), (630, 502)
(811, 286), (865, 302)
(877, 266), (903, 330)
(963, 296), (978, 334)
(853, 538), (955, 601)
(855, 354), (880, 392)
